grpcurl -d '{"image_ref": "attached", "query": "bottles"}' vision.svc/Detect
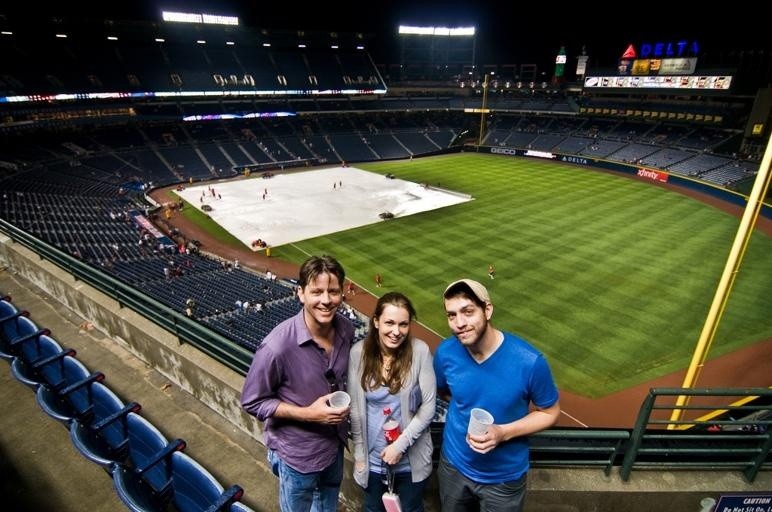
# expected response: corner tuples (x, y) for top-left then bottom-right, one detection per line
(382, 407), (401, 445)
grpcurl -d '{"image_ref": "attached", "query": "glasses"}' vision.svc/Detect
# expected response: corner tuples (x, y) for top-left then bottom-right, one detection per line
(323, 369), (339, 393)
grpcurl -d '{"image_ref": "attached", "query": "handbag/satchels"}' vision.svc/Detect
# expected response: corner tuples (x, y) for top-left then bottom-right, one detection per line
(409, 384), (421, 414)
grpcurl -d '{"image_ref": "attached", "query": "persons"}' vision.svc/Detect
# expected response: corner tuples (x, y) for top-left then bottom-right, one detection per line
(423, 276), (563, 512)
(239, 253), (358, 512)
(1, 67), (772, 335)
(342, 290), (442, 510)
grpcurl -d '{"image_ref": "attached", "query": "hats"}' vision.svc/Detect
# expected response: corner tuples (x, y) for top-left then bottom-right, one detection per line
(443, 279), (491, 303)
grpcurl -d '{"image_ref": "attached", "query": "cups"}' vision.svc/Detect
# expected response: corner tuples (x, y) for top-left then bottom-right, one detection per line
(328, 390), (351, 409)
(465, 407), (496, 446)
